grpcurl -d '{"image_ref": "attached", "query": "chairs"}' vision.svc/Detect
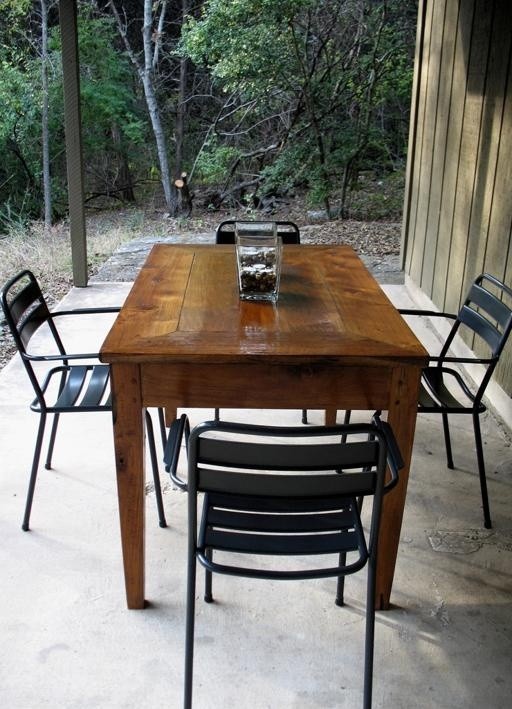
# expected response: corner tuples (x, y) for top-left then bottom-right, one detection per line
(165, 418), (404, 709)
(0, 270), (168, 530)
(214, 219), (308, 424)
(335, 272), (512, 529)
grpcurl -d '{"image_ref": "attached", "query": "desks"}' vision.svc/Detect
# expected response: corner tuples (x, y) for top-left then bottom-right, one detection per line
(107, 241), (430, 611)
